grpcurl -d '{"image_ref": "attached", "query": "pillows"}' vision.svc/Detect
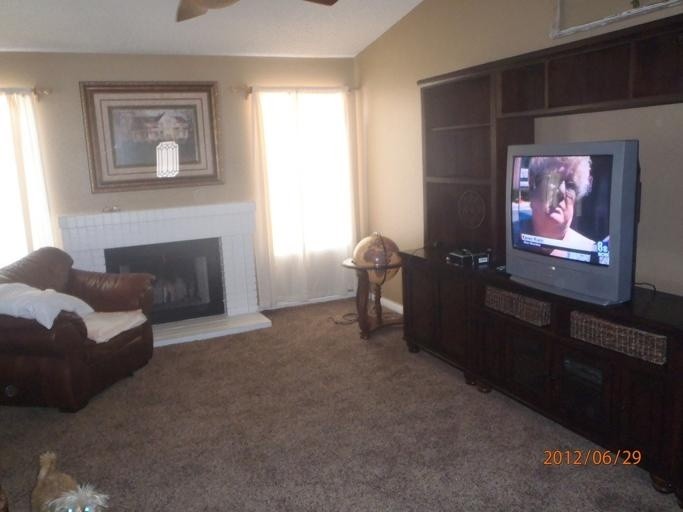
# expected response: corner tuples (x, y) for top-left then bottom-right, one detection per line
(0, 281), (97, 333)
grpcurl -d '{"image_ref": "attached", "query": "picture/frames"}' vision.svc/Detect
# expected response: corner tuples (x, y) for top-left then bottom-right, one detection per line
(78, 80), (226, 194)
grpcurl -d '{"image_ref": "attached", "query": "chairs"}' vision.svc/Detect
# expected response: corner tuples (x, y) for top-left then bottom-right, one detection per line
(0, 245), (157, 415)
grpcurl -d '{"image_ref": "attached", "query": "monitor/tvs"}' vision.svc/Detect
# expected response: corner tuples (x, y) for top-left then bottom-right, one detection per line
(505, 139), (642, 306)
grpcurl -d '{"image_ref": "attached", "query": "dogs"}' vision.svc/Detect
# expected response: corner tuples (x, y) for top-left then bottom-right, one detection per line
(30, 450), (110, 512)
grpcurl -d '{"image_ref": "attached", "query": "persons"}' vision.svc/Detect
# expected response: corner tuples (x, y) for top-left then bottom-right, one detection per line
(511, 155), (610, 265)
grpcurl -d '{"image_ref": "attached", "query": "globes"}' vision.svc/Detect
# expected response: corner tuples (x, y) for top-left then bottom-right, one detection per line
(341, 231), (401, 339)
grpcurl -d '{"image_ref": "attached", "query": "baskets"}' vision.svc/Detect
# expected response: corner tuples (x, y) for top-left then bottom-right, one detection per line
(484, 284), (553, 328)
(568, 310), (668, 366)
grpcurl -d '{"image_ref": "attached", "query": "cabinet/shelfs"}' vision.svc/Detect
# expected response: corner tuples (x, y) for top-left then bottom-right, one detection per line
(394, 250), (682, 508)
(416, 53), (534, 261)
(496, 14), (681, 119)
